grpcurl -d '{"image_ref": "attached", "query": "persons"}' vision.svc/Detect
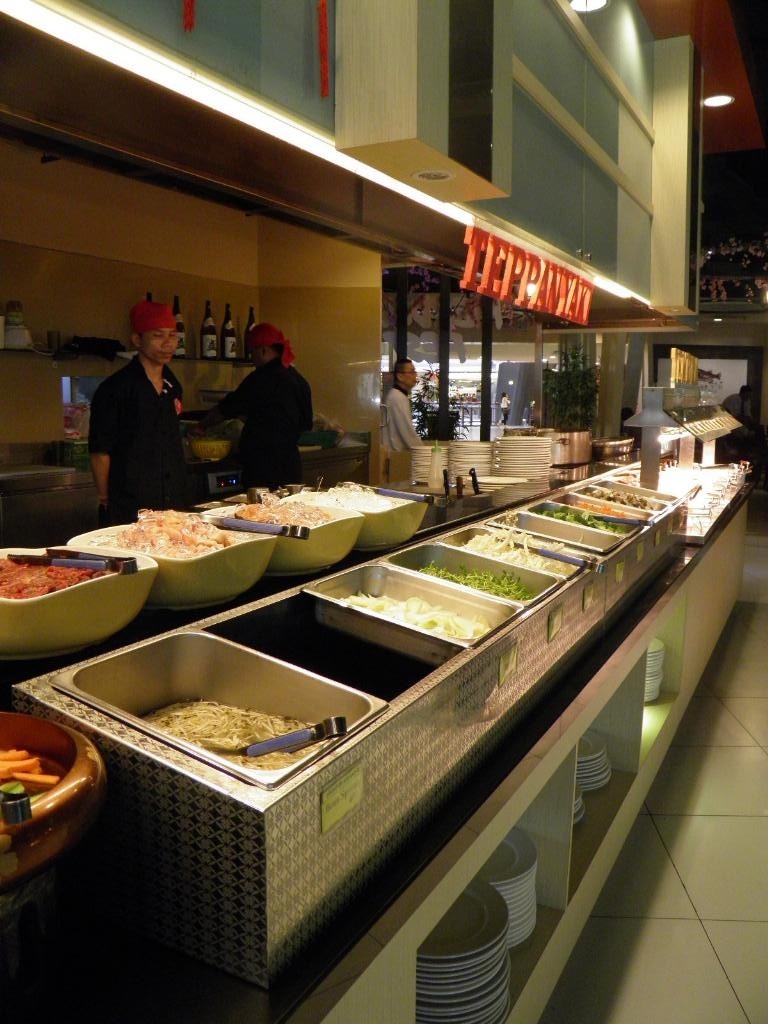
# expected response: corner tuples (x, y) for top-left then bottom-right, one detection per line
(381, 360), (424, 450)
(186, 322), (312, 490)
(497, 392), (511, 429)
(89, 302), (185, 526)
(722, 385), (753, 422)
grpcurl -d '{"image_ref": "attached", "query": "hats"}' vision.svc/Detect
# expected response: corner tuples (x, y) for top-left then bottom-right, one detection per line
(246, 324), (297, 370)
(130, 298), (176, 333)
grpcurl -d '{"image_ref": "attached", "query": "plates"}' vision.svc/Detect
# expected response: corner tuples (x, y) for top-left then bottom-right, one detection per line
(417, 823), (540, 1024)
(448, 436), (552, 492)
(409, 446), (448, 484)
(573, 726), (611, 822)
(492, 484), (549, 506)
(644, 638), (665, 704)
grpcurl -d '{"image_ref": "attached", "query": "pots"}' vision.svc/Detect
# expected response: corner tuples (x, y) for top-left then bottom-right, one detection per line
(536, 427), (592, 467)
(190, 439), (233, 461)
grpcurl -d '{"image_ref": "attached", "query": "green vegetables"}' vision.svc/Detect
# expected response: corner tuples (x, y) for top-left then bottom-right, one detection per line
(533, 505), (628, 535)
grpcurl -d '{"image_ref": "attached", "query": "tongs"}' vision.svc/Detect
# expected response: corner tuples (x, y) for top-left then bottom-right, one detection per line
(5, 545), (139, 576)
(198, 514), (311, 540)
(336, 481), (434, 505)
(495, 537), (608, 575)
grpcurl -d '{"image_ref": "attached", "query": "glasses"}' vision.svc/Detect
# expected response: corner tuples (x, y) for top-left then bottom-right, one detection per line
(399, 371), (417, 375)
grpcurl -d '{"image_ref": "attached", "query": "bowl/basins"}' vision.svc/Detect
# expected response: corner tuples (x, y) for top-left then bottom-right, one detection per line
(594, 438), (635, 460)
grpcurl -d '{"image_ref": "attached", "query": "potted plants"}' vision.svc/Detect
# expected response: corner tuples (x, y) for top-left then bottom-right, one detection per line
(542, 346), (601, 467)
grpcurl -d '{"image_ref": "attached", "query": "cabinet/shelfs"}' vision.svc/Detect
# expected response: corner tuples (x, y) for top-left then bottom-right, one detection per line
(293, 480), (754, 1022)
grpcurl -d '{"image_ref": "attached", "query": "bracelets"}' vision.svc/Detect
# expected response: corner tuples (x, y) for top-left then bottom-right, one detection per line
(98, 495), (107, 507)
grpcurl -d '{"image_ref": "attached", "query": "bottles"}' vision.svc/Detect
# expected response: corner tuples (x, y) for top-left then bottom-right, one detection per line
(244, 305), (256, 360)
(669, 346), (701, 409)
(172, 295), (186, 358)
(201, 299), (217, 359)
(429, 439), (444, 488)
(221, 303), (236, 359)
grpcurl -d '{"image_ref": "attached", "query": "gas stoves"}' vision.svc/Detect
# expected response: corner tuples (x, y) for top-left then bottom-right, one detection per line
(185, 460), (242, 496)
